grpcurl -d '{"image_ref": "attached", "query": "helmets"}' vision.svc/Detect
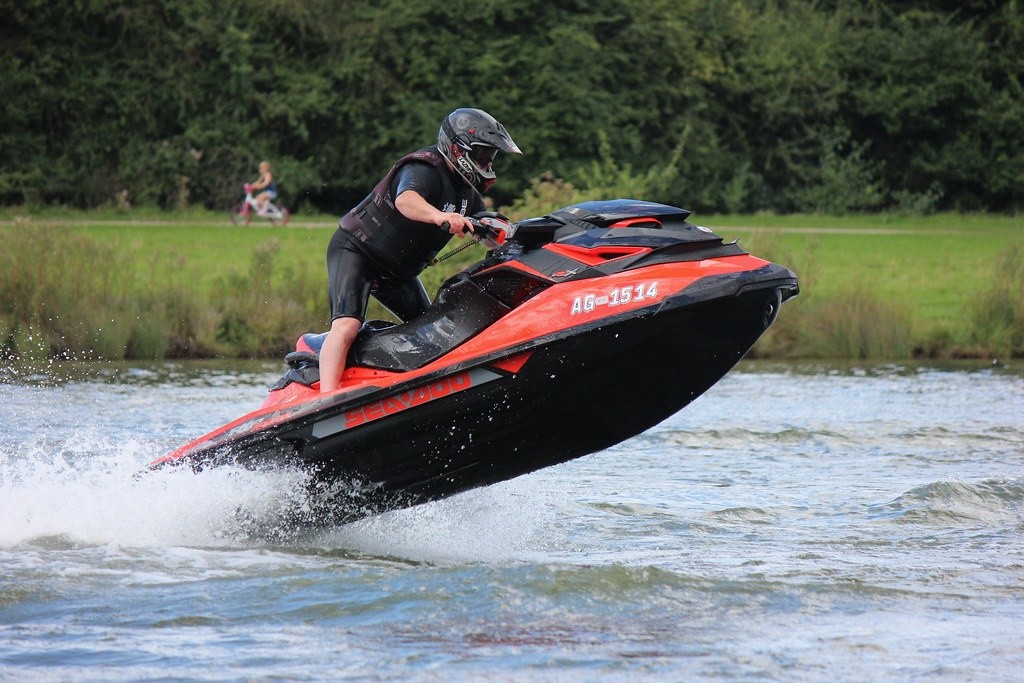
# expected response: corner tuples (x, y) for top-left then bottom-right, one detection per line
(438, 107), (524, 194)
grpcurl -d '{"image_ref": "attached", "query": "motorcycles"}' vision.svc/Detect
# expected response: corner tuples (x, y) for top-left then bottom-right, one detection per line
(138, 197), (800, 535)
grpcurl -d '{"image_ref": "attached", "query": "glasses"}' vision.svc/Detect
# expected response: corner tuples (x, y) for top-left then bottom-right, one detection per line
(470, 145), (499, 163)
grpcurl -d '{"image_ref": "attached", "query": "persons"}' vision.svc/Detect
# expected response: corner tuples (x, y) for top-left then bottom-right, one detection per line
(320, 108), (523, 392)
(253, 160), (277, 215)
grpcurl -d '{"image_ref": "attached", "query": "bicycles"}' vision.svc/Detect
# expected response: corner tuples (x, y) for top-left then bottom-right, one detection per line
(230, 182), (291, 229)
